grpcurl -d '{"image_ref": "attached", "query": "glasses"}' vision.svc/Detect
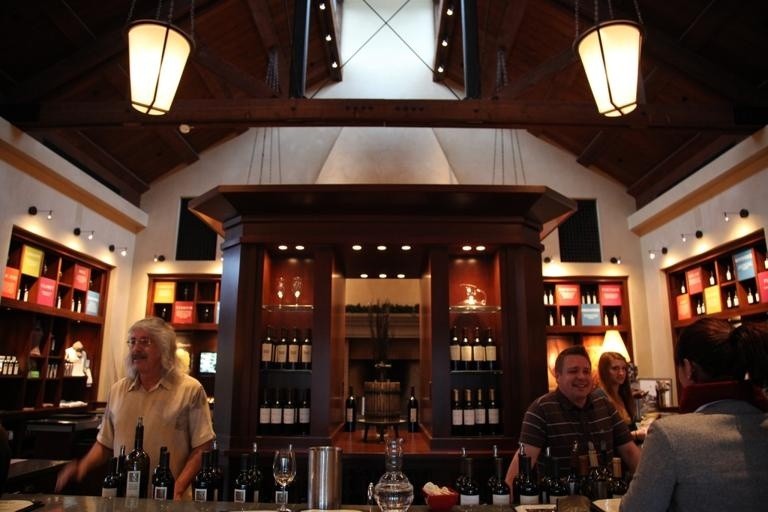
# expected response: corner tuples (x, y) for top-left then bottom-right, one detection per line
(127, 337), (156, 346)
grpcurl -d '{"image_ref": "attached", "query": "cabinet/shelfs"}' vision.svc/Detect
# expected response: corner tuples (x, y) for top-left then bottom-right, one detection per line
(187, 184), (578, 506)
(145, 273), (222, 422)
(659, 227), (768, 406)
(542, 275), (634, 393)
(0, 224), (117, 423)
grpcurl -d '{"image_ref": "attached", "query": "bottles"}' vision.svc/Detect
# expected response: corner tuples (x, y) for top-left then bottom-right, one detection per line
(702, 302), (706, 314)
(567, 440), (583, 495)
(182, 286), (189, 301)
(275, 327), (288, 370)
(734, 291), (740, 307)
(513, 442), (529, 505)
(17, 286), (22, 301)
(487, 388), (500, 435)
(726, 265), (732, 281)
(0, 355), (19, 377)
(460, 327), (472, 371)
(544, 290), (548, 305)
(747, 287), (754, 304)
(726, 292), (732, 308)
(561, 314), (566, 326)
(488, 446), (499, 505)
(193, 451), (213, 500)
(346, 386), (355, 432)
(582, 293), (585, 304)
(456, 447), (470, 505)
(159, 306), (166, 321)
(152, 445), (166, 497)
(571, 311), (575, 326)
(460, 458), (480, 505)
(154, 452), (173, 500)
(587, 292), (591, 304)
(261, 326), (275, 371)
(301, 329), (311, 369)
(541, 446), (555, 504)
(548, 460), (569, 503)
(696, 300), (702, 314)
(604, 312), (609, 326)
(549, 290), (554, 305)
(251, 443), (264, 503)
(57, 295), (63, 309)
(117, 444), (126, 499)
(71, 299), (75, 313)
(607, 457), (628, 499)
(451, 326), (461, 371)
(407, 386), (418, 433)
(374, 437), (414, 511)
(211, 441), (223, 501)
(23, 285), (29, 304)
(260, 389), (270, 436)
(298, 389), (310, 435)
(270, 388), (282, 435)
(587, 443), (602, 497)
(283, 388), (296, 435)
(76, 299), (83, 313)
(681, 280), (686, 294)
(592, 292), (597, 304)
(710, 270), (715, 285)
(476, 388), (487, 436)
(235, 453), (253, 503)
(102, 458), (120, 497)
(46, 360), (59, 378)
(463, 388), (476, 437)
(613, 312), (618, 326)
(755, 289), (760, 303)
(492, 458), (510, 504)
(123, 418), (149, 499)
(203, 307), (211, 324)
(549, 312), (554, 326)
(452, 389), (463, 437)
(473, 327), (486, 371)
(288, 327), (300, 370)
(520, 457), (539, 504)
(764, 256), (768, 269)
(485, 327), (497, 370)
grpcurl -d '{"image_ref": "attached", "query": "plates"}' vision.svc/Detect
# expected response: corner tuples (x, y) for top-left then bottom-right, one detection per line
(514, 503), (557, 512)
(593, 498), (621, 512)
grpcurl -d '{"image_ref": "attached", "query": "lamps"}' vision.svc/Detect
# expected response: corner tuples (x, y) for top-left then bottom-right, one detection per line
(648, 247), (667, 260)
(153, 255), (165, 262)
(723, 208), (749, 221)
(122, 0), (196, 117)
(29, 206), (54, 220)
(681, 230), (703, 242)
(75, 228), (96, 240)
(597, 330), (631, 364)
(544, 256), (555, 265)
(109, 244), (128, 257)
(573, 0), (647, 118)
(610, 256), (622, 264)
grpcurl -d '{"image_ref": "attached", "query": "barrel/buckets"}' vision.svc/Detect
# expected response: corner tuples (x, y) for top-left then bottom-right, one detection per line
(365, 381), (400, 421)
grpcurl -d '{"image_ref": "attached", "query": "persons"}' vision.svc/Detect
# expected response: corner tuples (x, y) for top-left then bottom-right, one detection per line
(64, 340), (92, 400)
(620, 320), (768, 511)
(505, 348), (641, 504)
(593, 353), (634, 430)
(56, 318), (214, 502)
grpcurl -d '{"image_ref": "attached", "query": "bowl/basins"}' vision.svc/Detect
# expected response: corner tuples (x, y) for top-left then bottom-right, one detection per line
(426, 487), (459, 511)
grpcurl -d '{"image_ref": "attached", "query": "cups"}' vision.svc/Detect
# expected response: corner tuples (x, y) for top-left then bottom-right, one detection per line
(271, 448), (298, 512)
(307, 445), (342, 509)
